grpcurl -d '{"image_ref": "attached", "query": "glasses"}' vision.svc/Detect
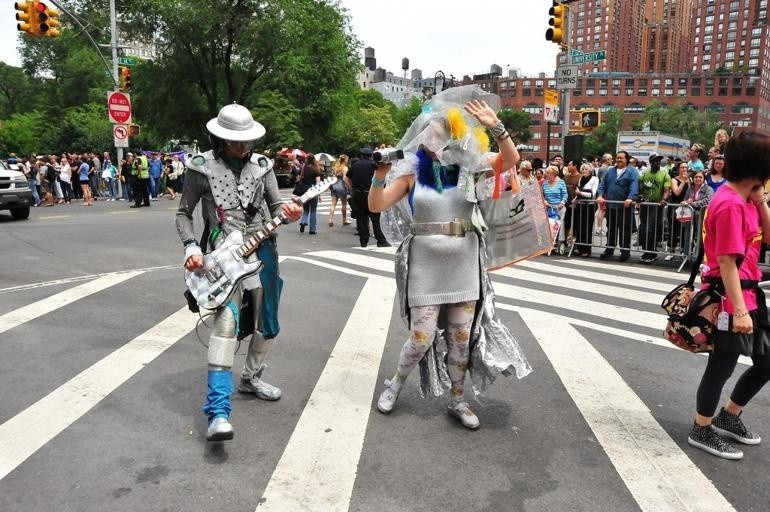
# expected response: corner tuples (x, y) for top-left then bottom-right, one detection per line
(527, 169), (532, 172)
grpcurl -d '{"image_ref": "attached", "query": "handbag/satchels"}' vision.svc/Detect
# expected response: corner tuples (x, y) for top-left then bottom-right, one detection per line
(675, 202), (693, 223)
(292, 174), (302, 195)
(168, 171), (177, 180)
(635, 205), (641, 228)
(662, 243), (747, 353)
(478, 153), (554, 272)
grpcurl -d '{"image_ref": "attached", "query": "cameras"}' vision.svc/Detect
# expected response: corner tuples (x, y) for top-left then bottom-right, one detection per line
(373, 146), (404, 164)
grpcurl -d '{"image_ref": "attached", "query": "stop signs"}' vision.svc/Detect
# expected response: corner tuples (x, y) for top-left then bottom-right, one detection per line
(107, 91), (132, 125)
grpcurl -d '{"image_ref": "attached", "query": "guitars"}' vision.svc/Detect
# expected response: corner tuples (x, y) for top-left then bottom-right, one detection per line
(184, 175), (338, 310)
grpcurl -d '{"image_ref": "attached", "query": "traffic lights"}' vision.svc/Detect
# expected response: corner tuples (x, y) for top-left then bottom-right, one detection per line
(579, 108), (601, 130)
(545, 3), (564, 44)
(121, 67), (131, 90)
(127, 125), (140, 136)
(15, 0), (59, 37)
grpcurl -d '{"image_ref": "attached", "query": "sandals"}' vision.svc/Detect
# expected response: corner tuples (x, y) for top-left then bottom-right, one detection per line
(170, 195), (176, 199)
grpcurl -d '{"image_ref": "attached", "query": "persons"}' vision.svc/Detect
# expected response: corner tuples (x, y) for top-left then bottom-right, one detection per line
(595, 153), (613, 238)
(163, 159), (178, 200)
(71, 155), (84, 200)
(76, 157), (93, 205)
(328, 154), (352, 228)
(36, 159), (55, 207)
(50, 156), (66, 204)
(172, 154), (184, 195)
(35, 161), (46, 203)
(587, 156), (599, 167)
(706, 153), (726, 195)
(684, 130), (770, 461)
(128, 151), (139, 201)
(708, 146), (721, 162)
(57, 157), (73, 204)
(595, 150), (641, 262)
(563, 159), (581, 247)
(130, 149), (151, 208)
(148, 153), (164, 201)
(635, 154), (672, 263)
(517, 160), (537, 191)
(681, 170), (715, 268)
(90, 152), (103, 197)
(541, 166), (569, 244)
(27, 158), (42, 208)
(665, 162), (692, 262)
(686, 143), (704, 172)
(554, 155), (570, 180)
(102, 151), (110, 171)
(535, 169), (545, 187)
(294, 153), (323, 235)
(289, 154), (303, 188)
(365, 100), (521, 429)
(104, 160), (119, 202)
(571, 163), (600, 259)
(160, 153), (169, 197)
(173, 103), (305, 443)
(343, 148), (392, 248)
(121, 156), (135, 203)
(714, 129), (729, 154)
(665, 156), (674, 173)
(84, 154), (100, 202)
(184, 153), (193, 174)
(15, 156), (30, 177)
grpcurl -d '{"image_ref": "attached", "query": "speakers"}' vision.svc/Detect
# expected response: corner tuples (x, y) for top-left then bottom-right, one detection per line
(564, 135), (584, 166)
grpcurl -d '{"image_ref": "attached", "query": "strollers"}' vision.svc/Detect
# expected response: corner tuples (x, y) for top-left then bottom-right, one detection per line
(545, 204), (565, 257)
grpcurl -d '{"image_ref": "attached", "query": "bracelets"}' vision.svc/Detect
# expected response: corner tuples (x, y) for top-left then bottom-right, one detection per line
(730, 310), (750, 321)
(368, 178), (386, 189)
(489, 121), (510, 146)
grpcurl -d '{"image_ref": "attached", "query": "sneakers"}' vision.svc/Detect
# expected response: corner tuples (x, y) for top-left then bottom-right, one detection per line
(378, 378), (399, 413)
(643, 253), (658, 263)
(237, 363), (281, 400)
(328, 222), (333, 226)
(33, 202), (40, 206)
(710, 406), (761, 444)
(582, 252), (589, 257)
(641, 254), (645, 261)
(343, 221), (351, 224)
(111, 199), (115, 201)
(665, 255), (675, 261)
(106, 199), (110, 201)
(44, 204), (52, 206)
(447, 402), (479, 429)
(206, 416), (234, 442)
(687, 419), (743, 459)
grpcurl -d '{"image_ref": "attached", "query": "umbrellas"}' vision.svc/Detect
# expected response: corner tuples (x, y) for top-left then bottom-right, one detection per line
(313, 152), (337, 175)
(280, 147), (307, 158)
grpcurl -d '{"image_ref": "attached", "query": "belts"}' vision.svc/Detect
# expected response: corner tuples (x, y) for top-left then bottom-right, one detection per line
(409, 217), (476, 236)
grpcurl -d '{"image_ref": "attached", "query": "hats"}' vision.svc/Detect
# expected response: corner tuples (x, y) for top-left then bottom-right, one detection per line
(520, 160), (533, 169)
(358, 148), (372, 155)
(205, 100), (266, 141)
(649, 154), (664, 162)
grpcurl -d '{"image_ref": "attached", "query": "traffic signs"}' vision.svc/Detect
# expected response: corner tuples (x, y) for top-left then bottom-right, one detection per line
(113, 125), (129, 148)
(543, 87), (559, 123)
(557, 65), (578, 89)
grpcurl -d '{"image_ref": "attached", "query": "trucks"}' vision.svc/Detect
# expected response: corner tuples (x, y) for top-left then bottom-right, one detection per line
(616, 131), (692, 164)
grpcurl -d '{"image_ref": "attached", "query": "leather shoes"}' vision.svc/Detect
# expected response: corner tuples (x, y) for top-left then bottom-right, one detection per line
(600, 248), (614, 258)
(300, 224), (304, 233)
(309, 231), (317, 234)
(377, 241), (392, 247)
(141, 204), (150, 206)
(130, 204), (139, 208)
(361, 242), (367, 247)
(619, 252), (630, 261)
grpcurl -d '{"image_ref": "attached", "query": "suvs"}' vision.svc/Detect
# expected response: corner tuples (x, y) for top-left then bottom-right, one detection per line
(0, 161), (32, 220)
